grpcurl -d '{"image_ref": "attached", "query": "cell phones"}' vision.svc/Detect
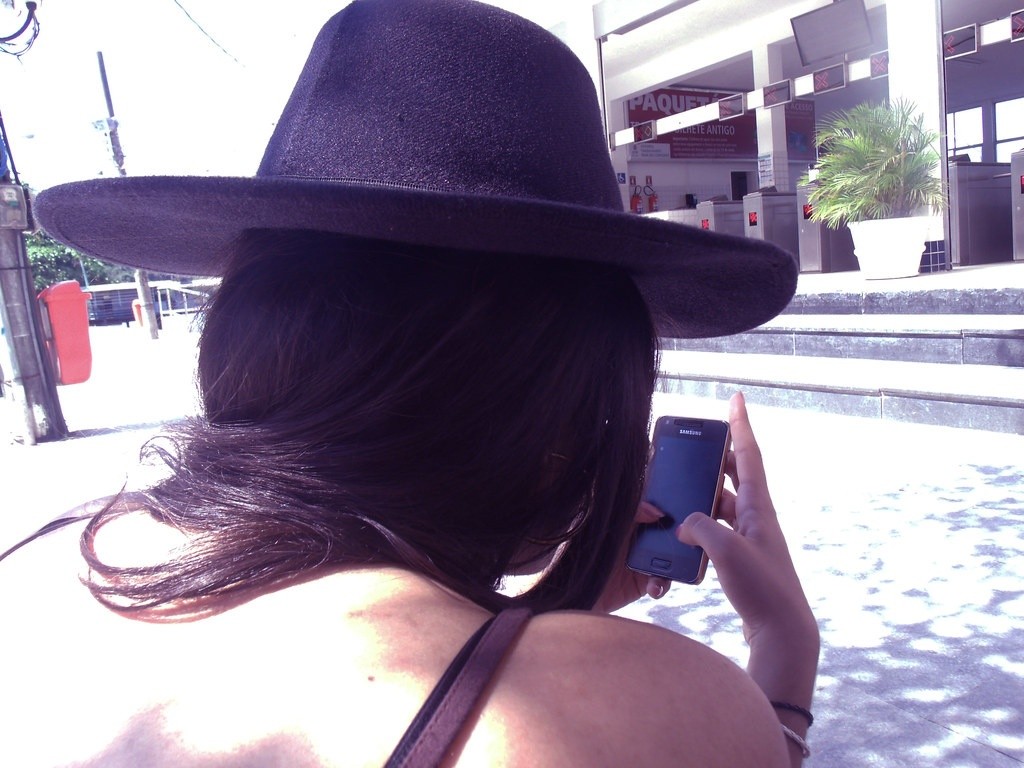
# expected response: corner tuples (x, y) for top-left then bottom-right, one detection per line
(625, 416), (732, 585)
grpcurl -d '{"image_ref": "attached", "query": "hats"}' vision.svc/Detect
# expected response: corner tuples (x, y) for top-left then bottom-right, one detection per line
(31, 0), (799, 339)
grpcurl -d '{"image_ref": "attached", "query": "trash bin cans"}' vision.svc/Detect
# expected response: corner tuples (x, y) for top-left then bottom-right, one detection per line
(37, 280), (93, 386)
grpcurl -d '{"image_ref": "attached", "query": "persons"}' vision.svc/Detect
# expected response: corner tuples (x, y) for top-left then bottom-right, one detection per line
(0, 1), (820, 768)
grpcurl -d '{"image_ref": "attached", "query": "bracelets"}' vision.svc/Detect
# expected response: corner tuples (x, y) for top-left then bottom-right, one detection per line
(780, 724), (811, 759)
(770, 701), (814, 728)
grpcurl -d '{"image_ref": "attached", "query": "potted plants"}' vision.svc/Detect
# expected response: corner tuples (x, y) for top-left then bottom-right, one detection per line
(794, 96), (952, 279)
